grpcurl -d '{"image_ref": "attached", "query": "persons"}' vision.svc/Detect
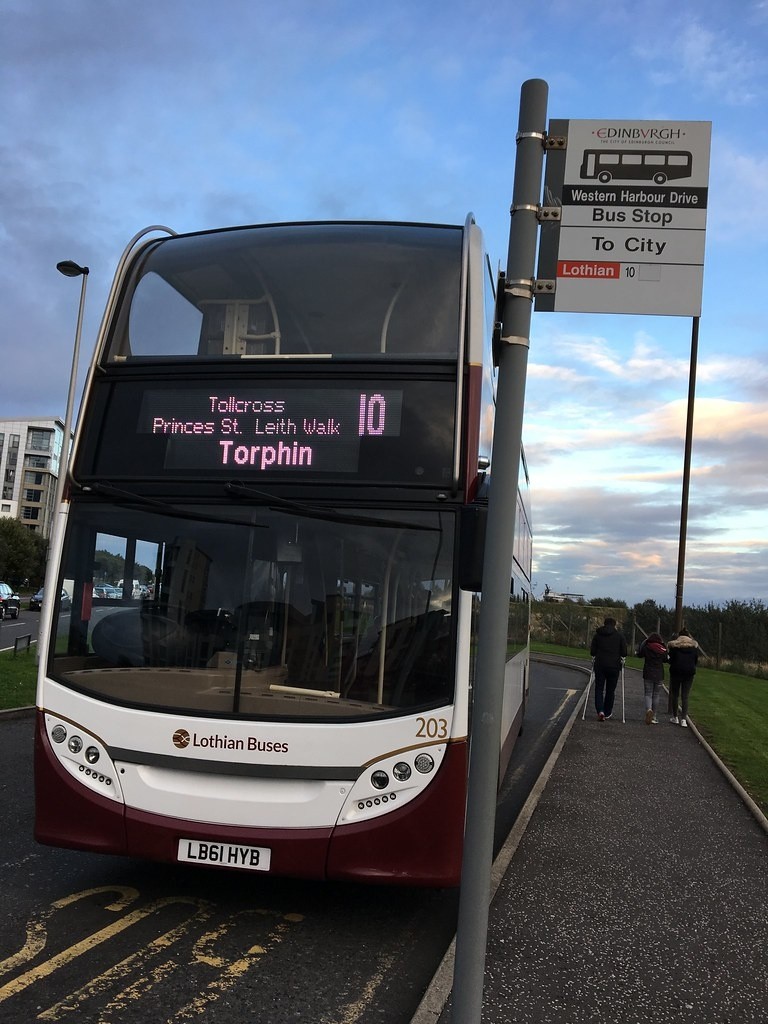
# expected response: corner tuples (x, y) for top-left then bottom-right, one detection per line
(666, 628), (698, 727)
(639, 633), (669, 725)
(591, 618), (628, 721)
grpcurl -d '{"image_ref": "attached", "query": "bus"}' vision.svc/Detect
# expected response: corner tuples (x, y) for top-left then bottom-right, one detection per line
(32, 211), (533, 890)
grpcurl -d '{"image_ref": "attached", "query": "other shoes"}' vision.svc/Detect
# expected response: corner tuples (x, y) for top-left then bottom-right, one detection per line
(599, 712), (606, 721)
(681, 719), (687, 727)
(651, 719), (658, 723)
(669, 716), (679, 724)
(606, 713), (612, 719)
(645, 709), (653, 724)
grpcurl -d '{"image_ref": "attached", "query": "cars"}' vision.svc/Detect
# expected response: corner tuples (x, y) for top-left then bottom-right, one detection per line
(94, 579), (154, 599)
(30, 587), (71, 612)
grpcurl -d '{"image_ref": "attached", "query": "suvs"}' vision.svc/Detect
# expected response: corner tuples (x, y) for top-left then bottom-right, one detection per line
(0, 581), (22, 621)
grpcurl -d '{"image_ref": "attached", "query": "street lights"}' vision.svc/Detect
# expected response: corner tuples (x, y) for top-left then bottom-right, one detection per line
(54, 260), (90, 519)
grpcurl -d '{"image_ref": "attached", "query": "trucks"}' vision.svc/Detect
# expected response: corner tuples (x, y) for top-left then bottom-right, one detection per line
(62, 579), (100, 604)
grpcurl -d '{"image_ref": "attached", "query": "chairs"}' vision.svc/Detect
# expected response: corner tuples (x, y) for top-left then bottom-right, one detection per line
(183, 611), (232, 652)
